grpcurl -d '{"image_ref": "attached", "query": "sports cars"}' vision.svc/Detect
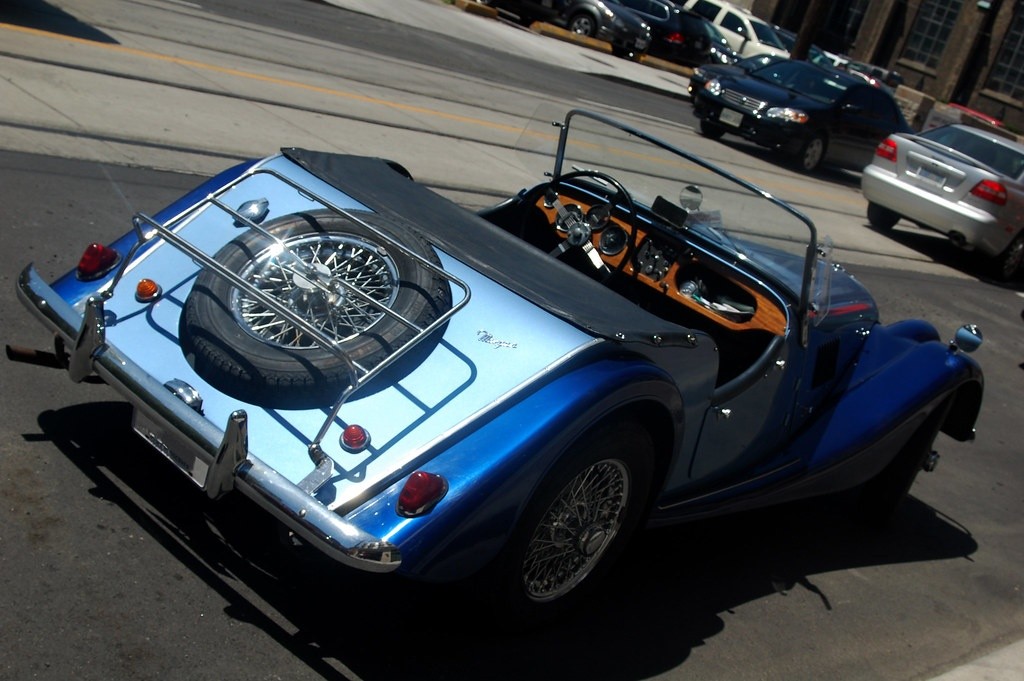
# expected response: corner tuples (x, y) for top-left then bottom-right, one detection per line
(16, 104), (988, 629)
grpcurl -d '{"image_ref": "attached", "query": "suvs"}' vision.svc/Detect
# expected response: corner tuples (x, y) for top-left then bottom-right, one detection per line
(608, 0), (736, 70)
(670, 0), (792, 61)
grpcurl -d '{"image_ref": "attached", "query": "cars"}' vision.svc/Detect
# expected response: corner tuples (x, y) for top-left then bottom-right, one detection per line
(688, 51), (796, 108)
(860, 119), (1024, 275)
(770, 27), (900, 103)
(692, 58), (911, 175)
(476, 0), (652, 58)
